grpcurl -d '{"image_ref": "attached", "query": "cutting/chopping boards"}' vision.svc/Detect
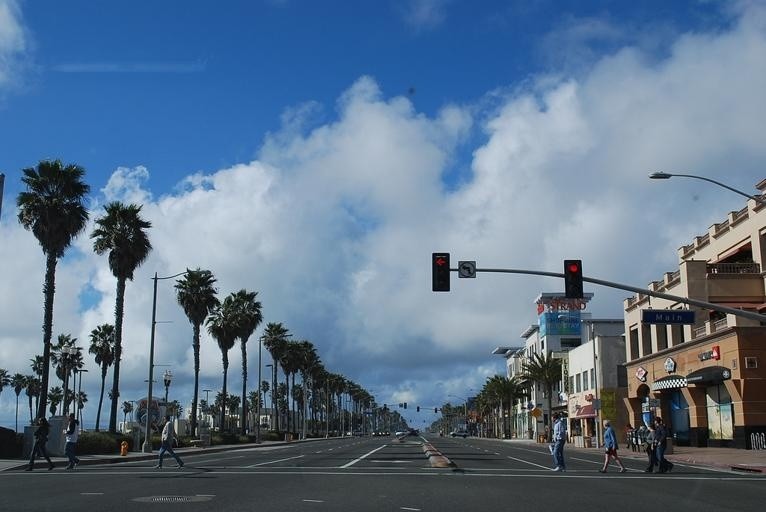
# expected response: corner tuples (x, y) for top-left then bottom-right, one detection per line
(120, 440), (128, 456)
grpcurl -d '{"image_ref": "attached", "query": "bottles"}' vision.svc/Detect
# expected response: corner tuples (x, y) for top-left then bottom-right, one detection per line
(67, 413), (75, 417)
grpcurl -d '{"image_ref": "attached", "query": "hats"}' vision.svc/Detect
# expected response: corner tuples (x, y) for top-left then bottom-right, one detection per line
(449, 430), (470, 438)
(371, 430), (382, 436)
(382, 431), (391, 437)
(395, 430), (404, 436)
(407, 429), (418, 436)
(346, 430), (363, 436)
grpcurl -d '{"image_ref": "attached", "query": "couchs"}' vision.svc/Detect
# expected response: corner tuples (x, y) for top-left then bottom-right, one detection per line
(432, 252), (451, 292)
(564, 258), (584, 297)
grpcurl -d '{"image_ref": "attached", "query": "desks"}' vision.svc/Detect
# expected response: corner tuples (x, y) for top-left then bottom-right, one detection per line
(75, 369), (88, 419)
(556, 313), (601, 448)
(164, 370), (173, 404)
(649, 171), (766, 208)
(447, 394), (467, 431)
(60, 340), (78, 416)
(255, 327), (291, 443)
(202, 389), (211, 423)
(139, 268), (213, 453)
(265, 364), (274, 431)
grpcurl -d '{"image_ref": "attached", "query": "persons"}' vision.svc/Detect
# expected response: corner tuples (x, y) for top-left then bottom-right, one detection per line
(25, 418), (56, 471)
(599, 420), (627, 473)
(643, 417), (673, 473)
(63, 413), (80, 470)
(551, 414), (566, 472)
(154, 416), (184, 469)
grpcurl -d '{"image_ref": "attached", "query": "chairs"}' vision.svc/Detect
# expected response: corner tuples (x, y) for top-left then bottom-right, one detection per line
(155, 465), (161, 468)
(177, 463), (183, 469)
(646, 462), (673, 474)
(48, 465), (55, 471)
(25, 468), (32, 470)
(75, 461), (80, 466)
(619, 469), (626, 472)
(554, 466), (563, 471)
(601, 470), (607, 473)
(66, 467), (73, 470)
